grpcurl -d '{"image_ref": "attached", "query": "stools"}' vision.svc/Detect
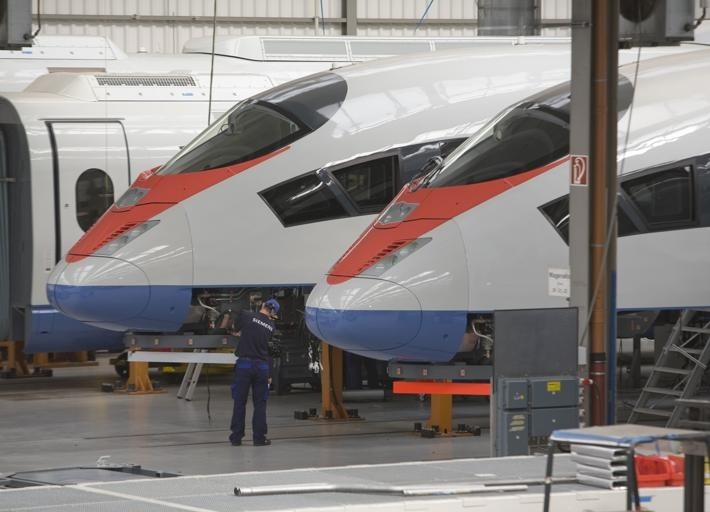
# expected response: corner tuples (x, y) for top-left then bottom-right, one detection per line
(542, 423), (709, 512)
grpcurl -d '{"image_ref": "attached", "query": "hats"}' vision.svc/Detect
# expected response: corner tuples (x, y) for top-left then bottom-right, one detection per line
(265, 299), (279, 312)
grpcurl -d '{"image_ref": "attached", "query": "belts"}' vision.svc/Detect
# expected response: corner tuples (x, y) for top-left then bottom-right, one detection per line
(239, 355), (263, 361)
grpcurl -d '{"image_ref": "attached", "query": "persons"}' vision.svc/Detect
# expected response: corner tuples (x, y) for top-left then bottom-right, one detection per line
(228, 298), (280, 446)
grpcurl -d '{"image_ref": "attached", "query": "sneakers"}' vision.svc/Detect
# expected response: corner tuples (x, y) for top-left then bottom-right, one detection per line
(254, 438), (271, 445)
(231, 439), (241, 445)
